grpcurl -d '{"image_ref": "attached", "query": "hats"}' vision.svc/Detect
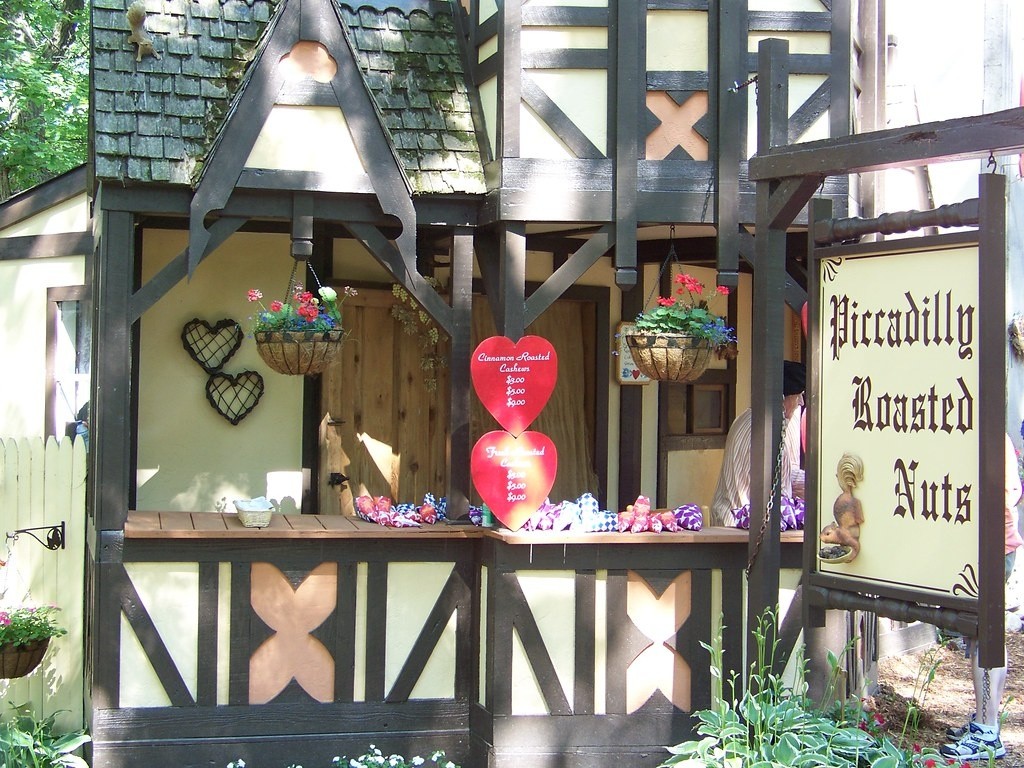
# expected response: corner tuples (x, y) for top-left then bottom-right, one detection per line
(783, 360), (806, 396)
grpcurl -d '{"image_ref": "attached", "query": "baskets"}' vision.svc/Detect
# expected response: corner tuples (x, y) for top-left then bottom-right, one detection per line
(234, 499), (276, 528)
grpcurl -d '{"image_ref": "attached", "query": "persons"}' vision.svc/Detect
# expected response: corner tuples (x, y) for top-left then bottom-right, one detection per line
(939, 429), (1024, 760)
(709, 360), (806, 529)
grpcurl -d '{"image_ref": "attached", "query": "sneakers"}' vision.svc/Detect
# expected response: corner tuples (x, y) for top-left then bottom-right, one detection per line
(946, 712), (977, 740)
(940, 722), (1006, 760)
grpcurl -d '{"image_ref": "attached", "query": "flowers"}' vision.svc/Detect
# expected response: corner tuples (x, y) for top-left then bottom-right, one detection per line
(0, 601), (68, 648)
(613, 272), (739, 359)
(244, 282), (359, 340)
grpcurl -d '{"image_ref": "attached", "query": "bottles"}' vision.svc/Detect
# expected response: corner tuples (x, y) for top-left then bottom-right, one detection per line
(75, 420), (89, 454)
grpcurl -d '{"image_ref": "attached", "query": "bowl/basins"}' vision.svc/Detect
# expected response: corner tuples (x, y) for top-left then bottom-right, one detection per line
(817, 545), (852, 563)
